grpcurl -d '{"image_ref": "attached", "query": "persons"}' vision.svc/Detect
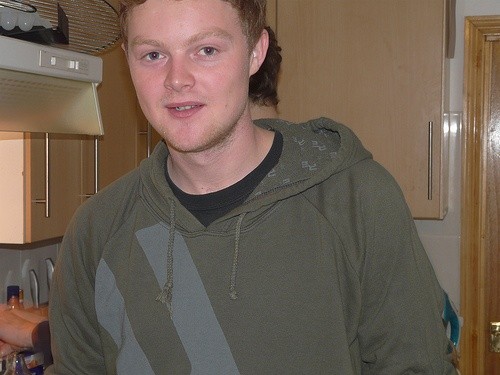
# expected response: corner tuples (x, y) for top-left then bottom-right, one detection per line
(44, 1), (458, 375)
(0, 27), (283, 375)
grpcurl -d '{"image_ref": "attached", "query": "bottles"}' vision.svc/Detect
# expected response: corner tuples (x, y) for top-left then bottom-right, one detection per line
(0, 286), (42, 375)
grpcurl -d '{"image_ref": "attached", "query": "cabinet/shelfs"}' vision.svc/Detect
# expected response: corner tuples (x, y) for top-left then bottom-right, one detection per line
(0, 0), (457, 245)
